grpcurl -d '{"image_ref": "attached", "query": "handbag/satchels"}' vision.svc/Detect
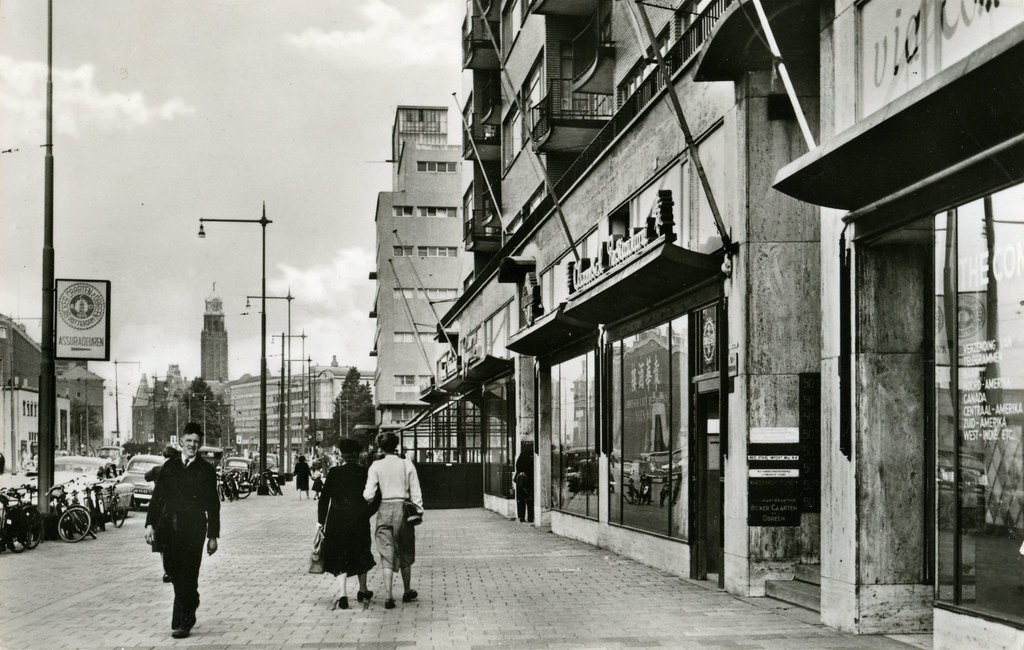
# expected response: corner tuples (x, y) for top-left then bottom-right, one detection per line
(403, 500), (422, 526)
(308, 527), (326, 574)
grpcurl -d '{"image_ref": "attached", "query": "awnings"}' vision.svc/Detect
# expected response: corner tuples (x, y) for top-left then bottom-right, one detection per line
(560, 232), (718, 324)
(505, 302), (591, 356)
(419, 354), (511, 405)
(769, 22), (1024, 211)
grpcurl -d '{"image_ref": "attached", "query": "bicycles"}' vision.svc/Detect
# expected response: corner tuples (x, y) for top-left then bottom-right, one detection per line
(46, 475), (106, 543)
(0, 484), (43, 553)
(216, 469), (252, 502)
(623, 470), (639, 504)
(262, 465), (283, 496)
(105, 480), (127, 528)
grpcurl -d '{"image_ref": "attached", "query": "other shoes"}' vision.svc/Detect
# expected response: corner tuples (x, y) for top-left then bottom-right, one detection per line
(172, 626), (191, 638)
(307, 497), (310, 499)
(298, 499), (301, 501)
(385, 599), (395, 609)
(403, 590), (417, 603)
(318, 497), (320, 499)
(163, 573), (172, 583)
(314, 498), (316, 500)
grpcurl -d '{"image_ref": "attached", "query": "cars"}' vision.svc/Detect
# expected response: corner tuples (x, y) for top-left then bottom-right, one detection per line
(224, 457), (259, 492)
(54, 456), (134, 516)
(99, 446), (127, 465)
(639, 452), (680, 482)
(565, 447), (597, 466)
(199, 446), (224, 466)
(122, 455), (166, 511)
(253, 454), (279, 474)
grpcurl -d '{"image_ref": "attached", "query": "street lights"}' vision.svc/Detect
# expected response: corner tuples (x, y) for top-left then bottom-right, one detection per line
(198, 200), (273, 495)
(271, 331), (307, 455)
(188, 390), (206, 423)
(292, 370), (316, 455)
(203, 400), (220, 446)
(113, 359), (142, 446)
(176, 390), (195, 444)
(246, 290), (295, 486)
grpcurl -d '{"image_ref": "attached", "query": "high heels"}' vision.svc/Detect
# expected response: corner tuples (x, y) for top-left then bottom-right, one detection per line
(339, 597), (348, 609)
(357, 591), (374, 603)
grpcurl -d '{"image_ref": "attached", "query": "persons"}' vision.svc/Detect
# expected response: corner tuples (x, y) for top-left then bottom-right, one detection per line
(144, 422), (220, 640)
(291, 455), (324, 500)
(551, 444), (568, 508)
(513, 449), (534, 523)
(317, 432), (425, 608)
